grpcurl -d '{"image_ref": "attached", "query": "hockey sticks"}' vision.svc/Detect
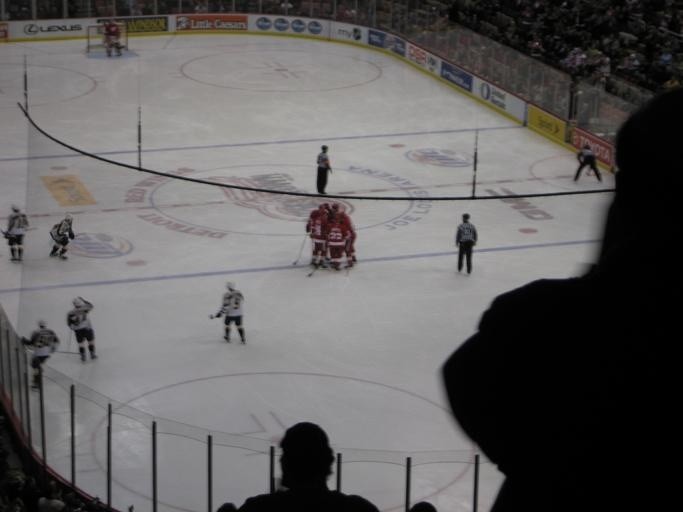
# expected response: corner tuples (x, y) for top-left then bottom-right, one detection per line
(308, 266), (318, 277)
(293, 235), (307, 265)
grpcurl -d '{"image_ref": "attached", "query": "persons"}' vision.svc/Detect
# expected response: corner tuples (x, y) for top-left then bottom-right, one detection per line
(49, 212), (73, 260)
(306, 202), (357, 269)
(313, 145), (331, 195)
(404, 2), (680, 136)
(1, 1), (405, 38)
(210, 279), (246, 344)
(19, 319), (61, 388)
(240, 422), (378, 512)
(454, 212), (479, 273)
(0, 451), (109, 512)
(101, 20), (126, 57)
(439, 80), (682, 507)
(3, 203), (28, 261)
(64, 295), (96, 361)
(575, 142), (605, 183)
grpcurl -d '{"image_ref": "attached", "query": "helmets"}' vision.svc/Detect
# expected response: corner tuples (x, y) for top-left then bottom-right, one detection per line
(39, 319), (48, 329)
(226, 280), (236, 289)
(12, 205), (21, 212)
(320, 203), (343, 221)
(322, 145), (328, 150)
(463, 213), (470, 219)
(65, 214), (73, 223)
(73, 297), (82, 308)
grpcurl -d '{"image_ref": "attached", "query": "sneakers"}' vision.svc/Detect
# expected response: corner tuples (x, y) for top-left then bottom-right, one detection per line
(50, 252), (68, 259)
(224, 336), (231, 342)
(311, 255), (355, 270)
(241, 337), (246, 342)
(81, 354), (97, 360)
(32, 383), (43, 389)
(11, 258), (24, 262)
